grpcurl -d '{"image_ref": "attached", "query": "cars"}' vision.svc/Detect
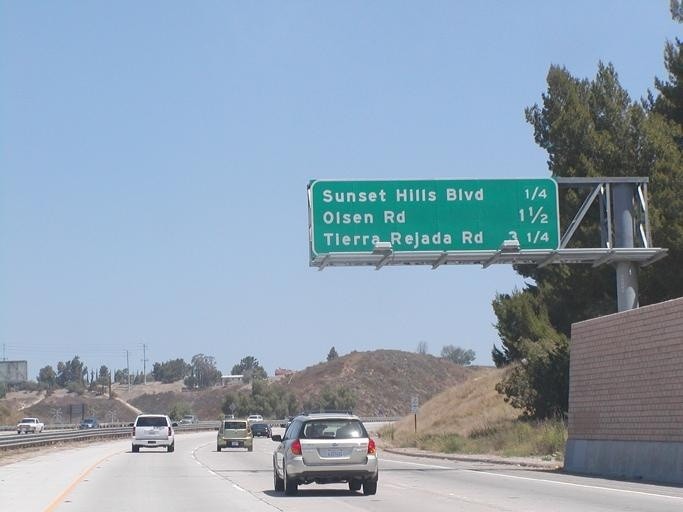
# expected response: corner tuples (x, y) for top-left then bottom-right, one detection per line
(181, 415), (198, 423)
(17, 418), (44, 434)
(250, 423), (273, 438)
(79, 417), (99, 429)
(217, 420), (253, 452)
(248, 415), (262, 422)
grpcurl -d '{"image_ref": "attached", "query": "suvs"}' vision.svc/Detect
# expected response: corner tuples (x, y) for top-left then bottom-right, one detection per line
(132, 413), (174, 453)
(274, 413), (379, 495)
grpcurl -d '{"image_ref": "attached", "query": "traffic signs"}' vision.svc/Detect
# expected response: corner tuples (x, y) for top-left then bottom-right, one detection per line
(312, 178), (560, 255)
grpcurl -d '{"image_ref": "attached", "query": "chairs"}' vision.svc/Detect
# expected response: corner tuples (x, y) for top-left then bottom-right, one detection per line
(306, 426), (356, 438)
